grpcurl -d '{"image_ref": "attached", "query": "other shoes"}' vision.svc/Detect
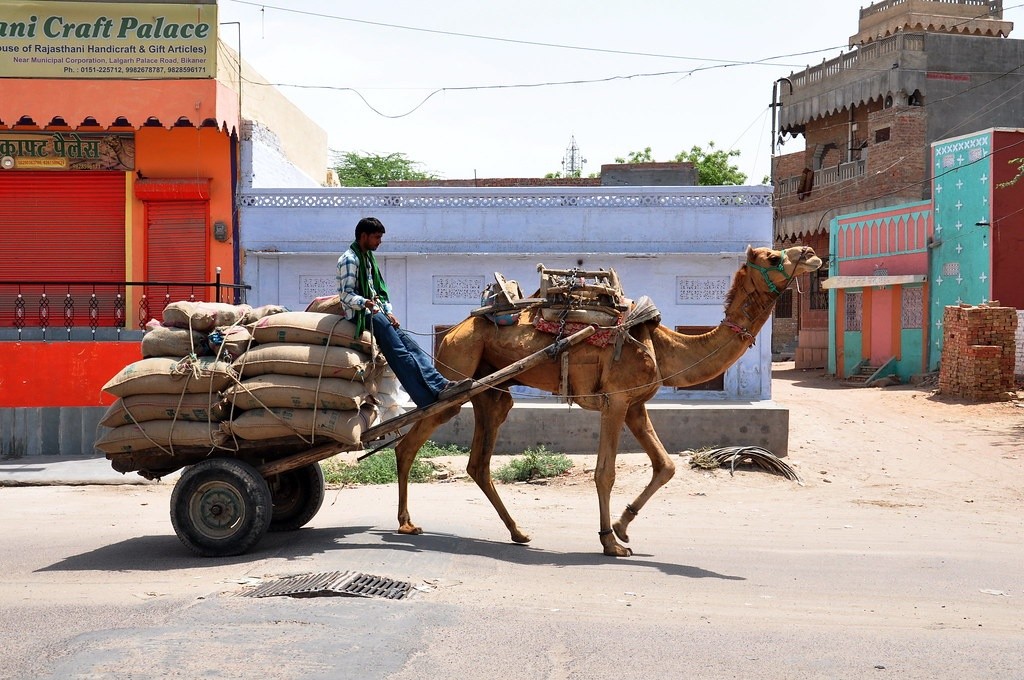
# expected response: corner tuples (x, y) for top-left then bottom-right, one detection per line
(438, 377), (473, 401)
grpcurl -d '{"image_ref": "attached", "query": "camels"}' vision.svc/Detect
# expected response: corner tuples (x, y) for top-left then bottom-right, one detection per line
(394, 242), (823, 557)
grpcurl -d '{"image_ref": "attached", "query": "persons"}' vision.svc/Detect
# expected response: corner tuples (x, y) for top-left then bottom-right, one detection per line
(337, 217), (473, 410)
